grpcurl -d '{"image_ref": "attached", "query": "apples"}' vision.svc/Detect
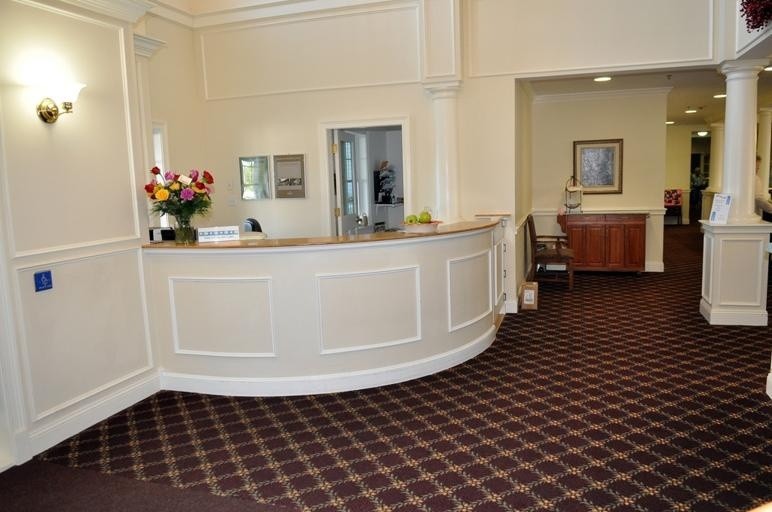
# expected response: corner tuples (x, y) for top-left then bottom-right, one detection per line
(418, 211), (433, 223)
(405, 215), (417, 225)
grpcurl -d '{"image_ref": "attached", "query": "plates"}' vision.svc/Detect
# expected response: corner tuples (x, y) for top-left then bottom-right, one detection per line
(401, 220), (444, 233)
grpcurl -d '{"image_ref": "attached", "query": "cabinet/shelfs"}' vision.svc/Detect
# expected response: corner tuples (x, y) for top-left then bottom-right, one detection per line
(566, 214), (646, 272)
(373, 204), (404, 233)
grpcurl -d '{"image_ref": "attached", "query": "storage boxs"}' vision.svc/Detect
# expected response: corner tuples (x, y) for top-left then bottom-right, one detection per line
(520, 281), (538, 311)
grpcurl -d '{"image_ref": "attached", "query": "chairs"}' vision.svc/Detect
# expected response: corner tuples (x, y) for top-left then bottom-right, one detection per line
(244, 217), (262, 232)
(527, 214), (574, 290)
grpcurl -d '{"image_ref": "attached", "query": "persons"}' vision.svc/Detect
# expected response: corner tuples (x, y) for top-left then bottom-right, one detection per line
(755, 155), (772, 218)
(689, 166), (707, 227)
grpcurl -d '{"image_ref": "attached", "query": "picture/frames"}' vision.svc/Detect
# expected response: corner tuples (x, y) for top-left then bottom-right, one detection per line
(574, 139), (622, 194)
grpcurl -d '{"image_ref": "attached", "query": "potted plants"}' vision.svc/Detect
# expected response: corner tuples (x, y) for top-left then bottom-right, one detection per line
(379, 161), (396, 204)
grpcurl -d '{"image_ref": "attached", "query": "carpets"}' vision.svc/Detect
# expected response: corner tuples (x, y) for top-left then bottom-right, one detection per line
(33, 224), (771, 512)
(0, 458), (294, 512)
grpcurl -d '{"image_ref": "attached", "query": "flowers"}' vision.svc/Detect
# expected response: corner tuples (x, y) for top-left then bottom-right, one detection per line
(143, 162), (214, 222)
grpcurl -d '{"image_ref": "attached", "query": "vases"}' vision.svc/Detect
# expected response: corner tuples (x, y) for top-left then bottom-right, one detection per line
(173, 214), (196, 244)
